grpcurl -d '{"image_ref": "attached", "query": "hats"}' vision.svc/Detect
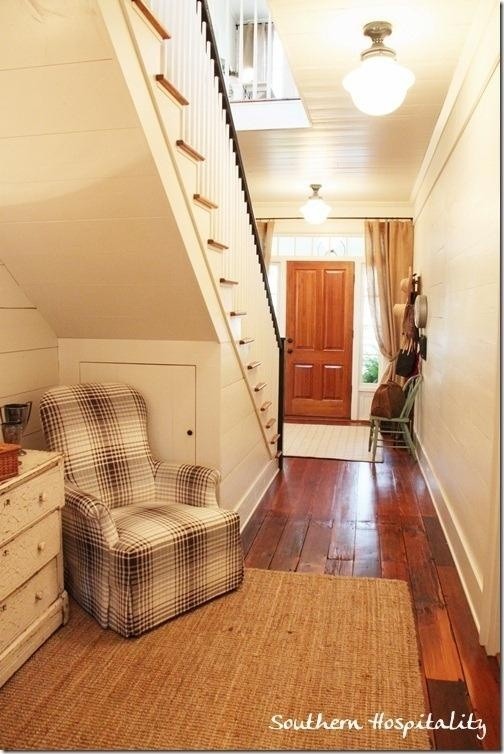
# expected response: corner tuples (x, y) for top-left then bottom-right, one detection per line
(393, 296), (411, 335)
(400, 266), (412, 304)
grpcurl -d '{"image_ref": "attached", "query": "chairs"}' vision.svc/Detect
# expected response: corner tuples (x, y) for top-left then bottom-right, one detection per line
(364, 373), (426, 467)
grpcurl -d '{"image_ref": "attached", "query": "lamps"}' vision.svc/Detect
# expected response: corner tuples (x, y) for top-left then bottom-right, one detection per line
(336, 2), (419, 124)
(298, 176), (334, 228)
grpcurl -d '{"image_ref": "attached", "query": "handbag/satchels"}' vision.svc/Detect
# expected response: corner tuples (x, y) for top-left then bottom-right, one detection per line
(371, 378), (405, 418)
(395, 335), (419, 378)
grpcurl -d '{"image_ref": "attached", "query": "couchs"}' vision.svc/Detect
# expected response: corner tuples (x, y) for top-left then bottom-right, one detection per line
(39, 379), (246, 643)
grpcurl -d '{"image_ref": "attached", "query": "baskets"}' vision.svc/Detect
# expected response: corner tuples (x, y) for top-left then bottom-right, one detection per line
(0, 443), (26, 480)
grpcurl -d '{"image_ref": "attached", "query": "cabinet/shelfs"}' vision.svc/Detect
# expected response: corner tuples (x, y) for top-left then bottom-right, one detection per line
(1, 440), (70, 697)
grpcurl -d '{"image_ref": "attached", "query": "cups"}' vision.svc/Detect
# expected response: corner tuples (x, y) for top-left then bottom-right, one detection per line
(0, 399), (32, 455)
(0, 421), (23, 464)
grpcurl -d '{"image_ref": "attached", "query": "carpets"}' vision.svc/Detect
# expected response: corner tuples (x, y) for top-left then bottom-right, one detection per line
(0, 565), (440, 748)
(280, 420), (383, 464)
